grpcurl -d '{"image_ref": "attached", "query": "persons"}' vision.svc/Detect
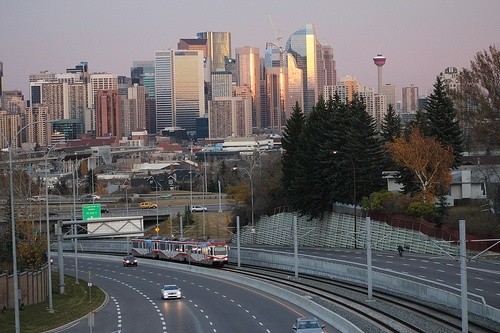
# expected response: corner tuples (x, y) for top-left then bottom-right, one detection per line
(397, 245), (405, 256)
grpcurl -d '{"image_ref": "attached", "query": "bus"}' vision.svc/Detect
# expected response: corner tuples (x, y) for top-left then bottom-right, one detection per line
(130, 238), (230, 268)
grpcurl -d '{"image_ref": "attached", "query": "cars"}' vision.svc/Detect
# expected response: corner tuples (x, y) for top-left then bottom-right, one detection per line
(191, 204), (207, 212)
(160, 285), (182, 300)
(139, 202), (157, 209)
(123, 256), (138, 267)
(292, 317), (326, 333)
(78, 195), (100, 201)
(26, 195), (46, 202)
(101, 208), (110, 214)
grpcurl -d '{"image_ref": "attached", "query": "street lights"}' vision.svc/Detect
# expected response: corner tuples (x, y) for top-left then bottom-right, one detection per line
(233, 167), (255, 245)
(331, 150), (357, 249)
(44, 141), (68, 311)
(76, 156), (100, 202)
(188, 171), (205, 212)
(8, 118), (83, 333)
